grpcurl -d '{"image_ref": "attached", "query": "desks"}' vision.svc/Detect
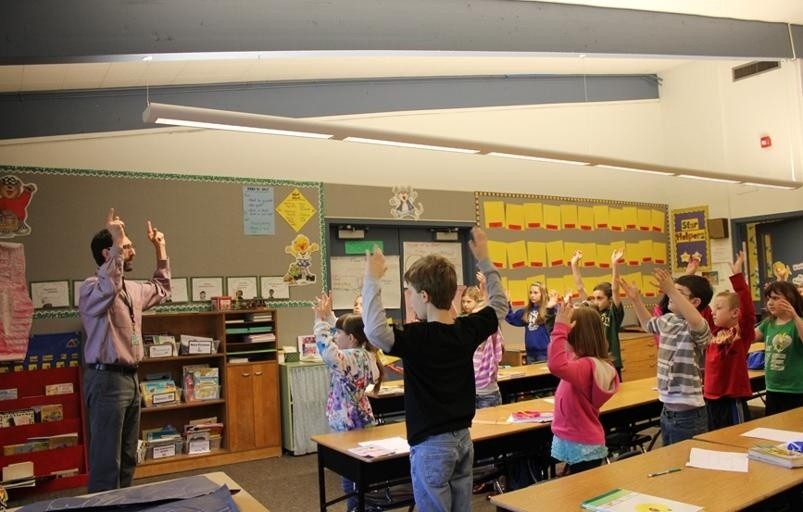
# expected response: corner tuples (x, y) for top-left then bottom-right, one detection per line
(0, 470), (268, 512)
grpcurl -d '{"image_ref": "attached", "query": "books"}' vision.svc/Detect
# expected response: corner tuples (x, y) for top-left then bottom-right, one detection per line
(581, 488), (704, 511)
(1, 376), (84, 491)
(746, 442), (803, 468)
(142, 312), (280, 461)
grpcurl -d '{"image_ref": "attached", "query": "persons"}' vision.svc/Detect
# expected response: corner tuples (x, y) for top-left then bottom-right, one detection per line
(236, 290), (243, 299)
(77, 206), (173, 495)
(312, 227), (510, 511)
(614, 251), (803, 447)
(268, 289), (274, 298)
(199, 291), (206, 301)
(504, 247), (624, 475)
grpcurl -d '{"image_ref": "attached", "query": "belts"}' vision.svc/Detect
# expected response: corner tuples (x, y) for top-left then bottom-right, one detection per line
(87, 362), (138, 374)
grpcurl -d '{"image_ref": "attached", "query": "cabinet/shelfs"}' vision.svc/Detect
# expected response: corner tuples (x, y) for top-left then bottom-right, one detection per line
(616, 329), (660, 382)
(0, 359), (91, 502)
(131, 351), (229, 478)
(210, 304), (283, 466)
(276, 361), (333, 457)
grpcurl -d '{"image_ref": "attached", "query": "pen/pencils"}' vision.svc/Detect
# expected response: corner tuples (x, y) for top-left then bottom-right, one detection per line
(648, 467), (684, 477)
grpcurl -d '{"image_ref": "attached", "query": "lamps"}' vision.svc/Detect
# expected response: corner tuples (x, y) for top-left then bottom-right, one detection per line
(137, 55), (802, 192)
